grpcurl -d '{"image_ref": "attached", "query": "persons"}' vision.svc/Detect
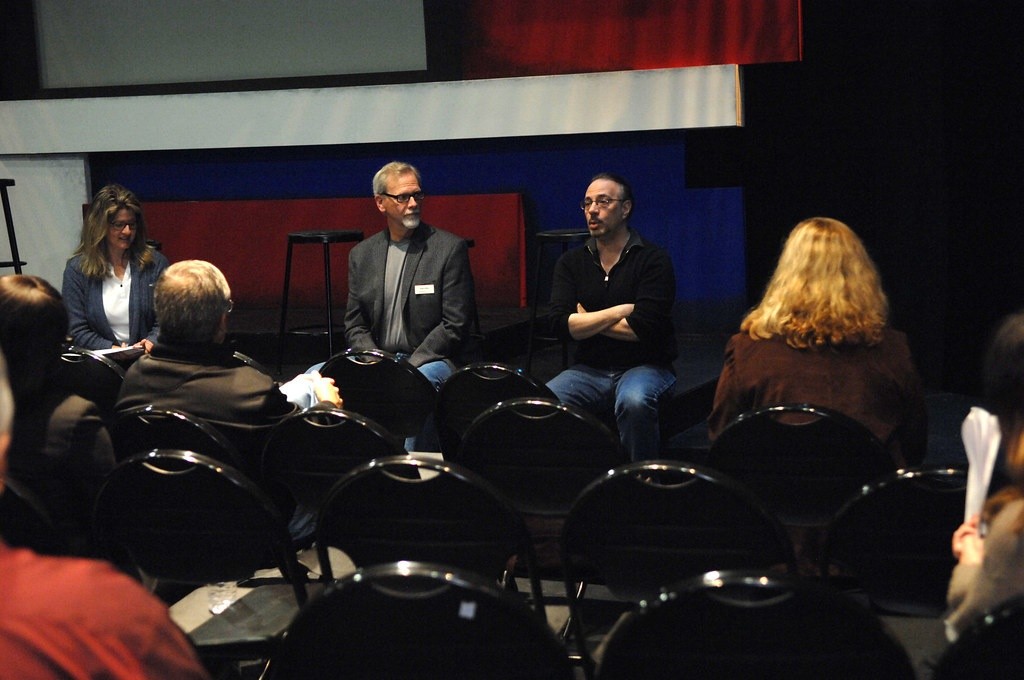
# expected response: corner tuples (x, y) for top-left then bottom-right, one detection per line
(708, 216), (928, 468)
(307, 161), (477, 448)
(0, 275), (115, 514)
(947, 313), (1024, 641)
(0, 352), (212, 680)
(547, 174), (682, 460)
(62, 182), (169, 370)
(116, 258), (343, 540)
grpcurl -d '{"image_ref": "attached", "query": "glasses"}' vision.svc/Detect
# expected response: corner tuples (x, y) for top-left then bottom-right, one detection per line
(110, 221), (138, 230)
(383, 191), (424, 203)
(224, 299), (234, 312)
(580, 198), (623, 210)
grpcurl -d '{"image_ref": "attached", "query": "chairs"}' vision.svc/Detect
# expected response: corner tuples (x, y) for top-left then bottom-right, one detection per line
(0, 343), (1023, 679)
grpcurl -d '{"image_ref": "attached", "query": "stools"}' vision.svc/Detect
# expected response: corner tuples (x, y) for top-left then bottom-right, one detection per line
(526, 230), (592, 373)
(464, 238), (488, 361)
(277, 231), (364, 374)
